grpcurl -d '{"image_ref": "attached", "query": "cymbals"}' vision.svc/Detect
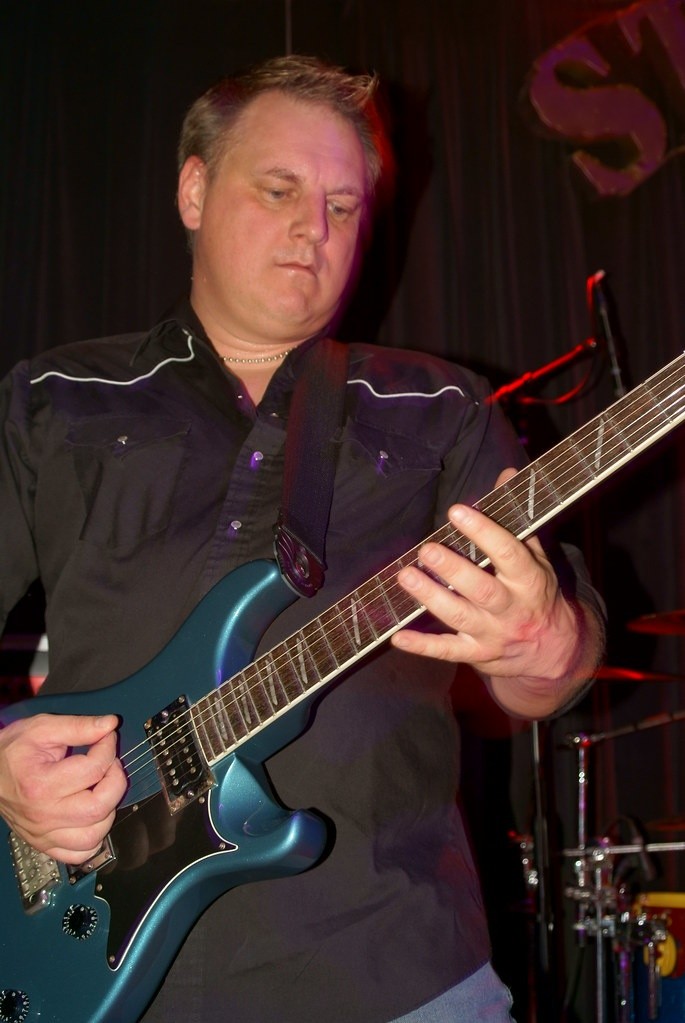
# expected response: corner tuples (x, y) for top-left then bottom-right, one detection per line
(591, 665), (682, 685)
(626, 608), (685, 636)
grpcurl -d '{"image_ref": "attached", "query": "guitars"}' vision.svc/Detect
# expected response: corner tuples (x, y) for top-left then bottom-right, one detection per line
(0, 344), (685, 1023)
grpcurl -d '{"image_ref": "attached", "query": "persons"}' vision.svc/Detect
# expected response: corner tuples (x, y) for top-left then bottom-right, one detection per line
(2, 55), (608, 1023)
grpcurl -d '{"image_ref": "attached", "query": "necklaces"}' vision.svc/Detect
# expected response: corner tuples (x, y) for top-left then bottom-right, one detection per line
(221, 345), (300, 364)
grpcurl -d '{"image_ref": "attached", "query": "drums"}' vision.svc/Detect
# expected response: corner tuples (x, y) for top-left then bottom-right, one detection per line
(600, 910), (670, 1023)
